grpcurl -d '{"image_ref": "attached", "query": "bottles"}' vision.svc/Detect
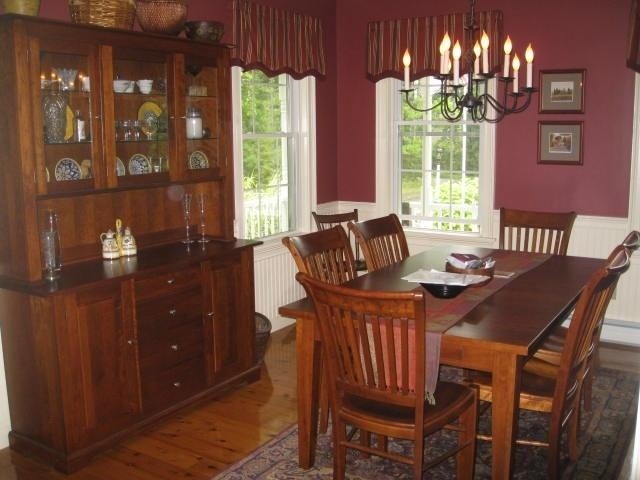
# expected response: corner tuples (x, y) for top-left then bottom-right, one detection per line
(39, 207), (65, 281)
(70, 110), (87, 144)
(41, 82), (68, 143)
(186, 107), (203, 139)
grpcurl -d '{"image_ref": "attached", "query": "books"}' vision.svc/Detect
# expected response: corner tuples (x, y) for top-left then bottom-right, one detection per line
(447, 253), (481, 270)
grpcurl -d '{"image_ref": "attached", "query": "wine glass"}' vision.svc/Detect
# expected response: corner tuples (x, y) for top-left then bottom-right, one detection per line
(112, 118), (159, 141)
(177, 191), (214, 245)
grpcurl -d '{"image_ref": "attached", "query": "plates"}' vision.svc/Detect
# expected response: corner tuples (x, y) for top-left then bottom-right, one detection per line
(136, 101), (163, 140)
(422, 281), (466, 299)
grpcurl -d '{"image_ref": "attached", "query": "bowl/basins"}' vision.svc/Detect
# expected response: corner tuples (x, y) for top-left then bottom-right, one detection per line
(124, 81), (134, 92)
(113, 79), (131, 93)
(445, 262), (494, 287)
(184, 20), (224, 42)
(44, 149), (210, 183)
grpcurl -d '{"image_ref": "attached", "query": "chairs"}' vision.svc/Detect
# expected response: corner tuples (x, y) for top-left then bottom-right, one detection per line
(533, 230), (640, 411)
(499, 206), (577, 255)
(283, 225), (387, 459)
(312, 208), (367, 279)
(295, 272), (477, 480)
(462, 249), (630, 480)
(347, 212), (409, 273)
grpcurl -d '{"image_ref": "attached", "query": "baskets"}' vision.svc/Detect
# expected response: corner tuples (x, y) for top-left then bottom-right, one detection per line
(133, 0), (188, 36)
(67, 0), (137, 32)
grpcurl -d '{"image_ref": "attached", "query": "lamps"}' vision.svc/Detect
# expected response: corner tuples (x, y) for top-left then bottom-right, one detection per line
(397, 0), (540, 124)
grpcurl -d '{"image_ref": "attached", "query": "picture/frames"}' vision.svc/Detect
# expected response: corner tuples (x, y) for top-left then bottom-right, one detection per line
(538, 69), (586, 113)
(537, 120), (584, 165)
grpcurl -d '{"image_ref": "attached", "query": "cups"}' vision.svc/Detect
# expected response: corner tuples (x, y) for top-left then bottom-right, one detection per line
(81, 76), (91, 92)
(53, 65), (78, 89)
(136, 79), (154, 95)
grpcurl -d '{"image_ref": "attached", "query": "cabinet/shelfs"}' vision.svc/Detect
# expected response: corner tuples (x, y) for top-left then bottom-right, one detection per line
(0, 244), (260, 474)
(1, 14), (232, 198)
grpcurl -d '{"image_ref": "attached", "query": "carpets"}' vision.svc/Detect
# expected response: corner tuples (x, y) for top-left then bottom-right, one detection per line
(208, 367), (639, 480)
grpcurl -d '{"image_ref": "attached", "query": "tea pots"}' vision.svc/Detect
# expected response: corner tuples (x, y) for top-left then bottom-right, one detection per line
(120, 226), (139, 257)
(99, 230), (122, 260)
(104, 261), (122, 279)
(120, 256), (138, 274)
